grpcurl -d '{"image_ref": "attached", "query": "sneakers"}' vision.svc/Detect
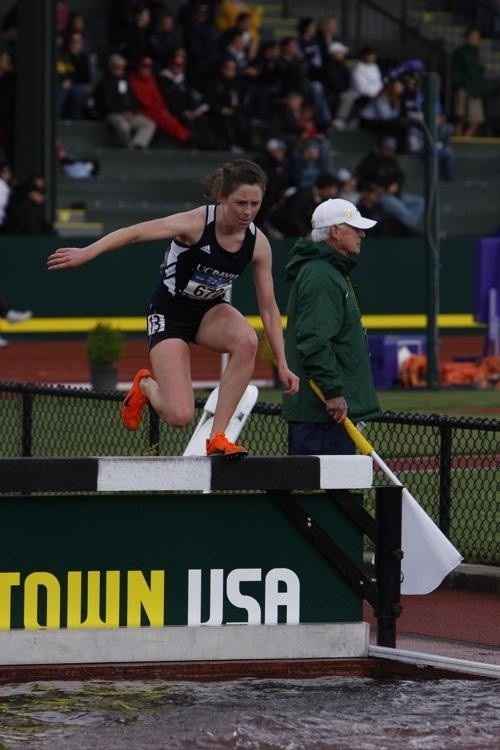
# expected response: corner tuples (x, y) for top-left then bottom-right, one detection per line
(122, 368), (152, 431)
(206, 432), (250, 458)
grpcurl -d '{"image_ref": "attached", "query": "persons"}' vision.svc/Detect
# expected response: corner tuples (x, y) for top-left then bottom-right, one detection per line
(270, 196), (386, 455)
(0, 0), (499, 241)
(44, 156), (301, 461)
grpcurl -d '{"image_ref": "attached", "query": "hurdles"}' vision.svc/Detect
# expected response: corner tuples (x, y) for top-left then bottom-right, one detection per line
(0, 455), (403, 683)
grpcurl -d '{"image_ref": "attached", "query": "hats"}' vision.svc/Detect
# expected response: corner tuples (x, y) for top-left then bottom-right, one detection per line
(327, 41), (349, 55)
(138, 57), (154, 66)
(311, 198), (378, 229)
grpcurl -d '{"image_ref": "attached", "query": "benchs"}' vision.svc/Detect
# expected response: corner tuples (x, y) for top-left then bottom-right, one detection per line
(44, 0), (500, 241)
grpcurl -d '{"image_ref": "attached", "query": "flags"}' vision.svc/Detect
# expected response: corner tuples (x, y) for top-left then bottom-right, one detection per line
(371, 488), (465, 600)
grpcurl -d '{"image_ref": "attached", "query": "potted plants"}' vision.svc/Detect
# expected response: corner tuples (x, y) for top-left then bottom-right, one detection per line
(87, 320), (123, 391)
(261, 333), (286, 389)
